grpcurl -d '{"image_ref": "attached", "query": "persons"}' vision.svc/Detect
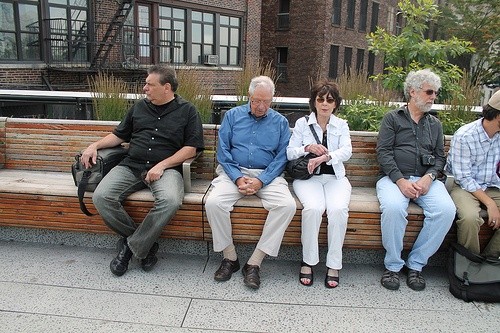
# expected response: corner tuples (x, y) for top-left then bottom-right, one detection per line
(287, 77), (352, 289)
(444, 90), (500, 303)
(205, 74), (297, 290)
(376, 68), (456, 291)
(81, 64), (205, 277)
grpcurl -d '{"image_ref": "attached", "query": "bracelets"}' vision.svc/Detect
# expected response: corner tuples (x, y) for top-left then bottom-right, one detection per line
(309, 143), (315, 154)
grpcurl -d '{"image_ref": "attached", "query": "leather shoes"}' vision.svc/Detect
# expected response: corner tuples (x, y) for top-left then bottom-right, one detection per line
(141, 242), (160, 270)
(110, 240), (133, 276)
(242, 259), (260, 289)
(215, 256), (239, 281)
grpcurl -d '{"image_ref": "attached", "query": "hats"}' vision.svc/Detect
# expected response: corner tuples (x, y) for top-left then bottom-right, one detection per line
(487, 90), (500, 110)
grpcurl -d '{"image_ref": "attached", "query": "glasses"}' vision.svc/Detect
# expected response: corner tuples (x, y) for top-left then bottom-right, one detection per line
(249, 94), (272, 106)
(419, 88), (440, 96)
(316, 96), (337, 103)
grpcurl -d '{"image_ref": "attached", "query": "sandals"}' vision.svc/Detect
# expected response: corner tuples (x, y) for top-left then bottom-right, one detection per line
(299, 260), (314, 286)
(325, 267), (340, 288)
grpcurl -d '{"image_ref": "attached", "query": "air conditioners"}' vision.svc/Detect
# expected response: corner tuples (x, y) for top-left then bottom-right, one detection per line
(204, 55), (219, 66)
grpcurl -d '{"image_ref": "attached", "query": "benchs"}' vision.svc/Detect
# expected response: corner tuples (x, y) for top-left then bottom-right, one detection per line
(0, 116), (495, 253)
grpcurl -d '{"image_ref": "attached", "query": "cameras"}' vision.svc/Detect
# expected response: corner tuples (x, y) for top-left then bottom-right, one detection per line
(421, 155), (436, 166)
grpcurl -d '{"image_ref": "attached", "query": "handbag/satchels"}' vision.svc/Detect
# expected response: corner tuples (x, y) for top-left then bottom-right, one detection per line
(448, 238), (500, 302)
(72, 144), (126, 192)
(285, 117), (321, 180)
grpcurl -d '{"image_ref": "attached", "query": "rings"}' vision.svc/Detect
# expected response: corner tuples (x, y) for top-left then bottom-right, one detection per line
(492, 221), (495, 224)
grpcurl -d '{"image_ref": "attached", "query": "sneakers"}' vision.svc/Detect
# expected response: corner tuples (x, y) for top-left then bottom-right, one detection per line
(381, 268), (399, 290)
(406, 266), (426, 290)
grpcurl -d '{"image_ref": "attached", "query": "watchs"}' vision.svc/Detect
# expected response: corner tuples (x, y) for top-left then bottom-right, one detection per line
(427, 174), (435, 180)
